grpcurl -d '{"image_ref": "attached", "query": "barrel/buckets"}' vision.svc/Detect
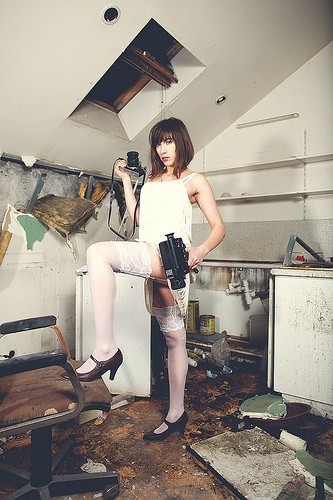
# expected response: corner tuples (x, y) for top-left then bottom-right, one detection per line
(248, 314), (268, 347)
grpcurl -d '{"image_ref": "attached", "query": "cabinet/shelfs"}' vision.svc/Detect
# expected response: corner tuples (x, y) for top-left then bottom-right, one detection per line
(266, 266), (333, 420)
(196, 151), (333, 203)
(74, 266), (154, 398)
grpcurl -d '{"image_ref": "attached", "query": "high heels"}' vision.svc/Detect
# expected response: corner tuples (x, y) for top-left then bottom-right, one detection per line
(143, 411), (189, 443)
(60, 346), (124, 383)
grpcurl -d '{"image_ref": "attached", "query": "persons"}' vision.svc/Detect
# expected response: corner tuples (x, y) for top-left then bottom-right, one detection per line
(58, 116), (226, 442)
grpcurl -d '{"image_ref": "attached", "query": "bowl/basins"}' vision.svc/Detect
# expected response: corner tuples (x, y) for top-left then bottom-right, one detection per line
(252, 402), (311, 433)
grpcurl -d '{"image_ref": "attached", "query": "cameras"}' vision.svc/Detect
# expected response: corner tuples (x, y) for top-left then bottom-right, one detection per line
(120, 151), (146, 177)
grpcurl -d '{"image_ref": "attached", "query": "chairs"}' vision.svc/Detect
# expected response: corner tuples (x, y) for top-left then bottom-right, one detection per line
(0, 315), (120, 500)
